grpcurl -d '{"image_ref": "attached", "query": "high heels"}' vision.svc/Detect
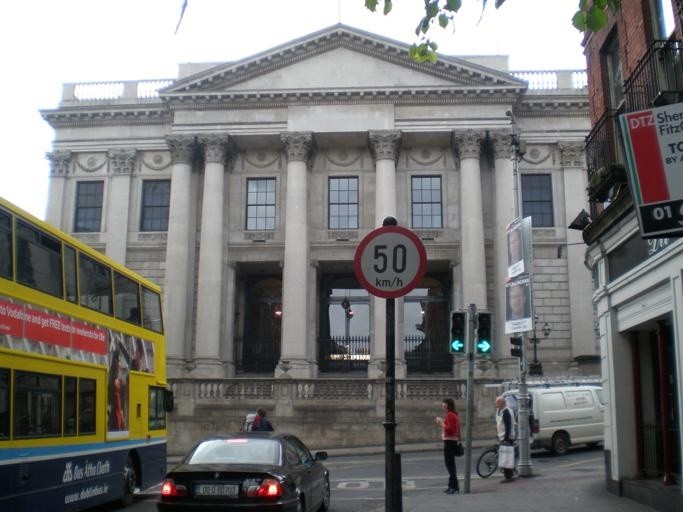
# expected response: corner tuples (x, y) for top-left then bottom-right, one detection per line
(443, 487), (461, 494)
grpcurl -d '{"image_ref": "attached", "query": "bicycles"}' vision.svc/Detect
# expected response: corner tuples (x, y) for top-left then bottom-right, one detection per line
(476, 446), (504, 478)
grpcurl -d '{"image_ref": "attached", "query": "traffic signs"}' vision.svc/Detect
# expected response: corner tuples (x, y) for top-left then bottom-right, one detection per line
(353, 225), (427, 299)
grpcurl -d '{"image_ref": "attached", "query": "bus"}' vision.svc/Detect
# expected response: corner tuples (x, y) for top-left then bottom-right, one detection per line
(0, 197), (174, 512)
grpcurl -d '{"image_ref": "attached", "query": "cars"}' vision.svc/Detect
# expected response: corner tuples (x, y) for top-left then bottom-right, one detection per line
(157, 431), (330, 512)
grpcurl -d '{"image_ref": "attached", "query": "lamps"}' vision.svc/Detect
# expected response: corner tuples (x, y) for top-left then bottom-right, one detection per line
(341, 267), (350, 310)
(346, 273), (354, 319)
(567, 209), (594, 231)
(651, 90), (680, 107)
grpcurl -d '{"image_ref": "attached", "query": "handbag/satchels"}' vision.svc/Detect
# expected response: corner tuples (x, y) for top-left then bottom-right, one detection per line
(455, 441), (465, 457)
(495, 444), (517, 472)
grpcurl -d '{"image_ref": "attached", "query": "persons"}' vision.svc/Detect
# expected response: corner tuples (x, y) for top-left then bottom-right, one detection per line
(108, 351), (125, 431)
(114, 335), (144, 371)
(506, 230), (523, 267)
(251, 408), (274, 432)
(506, 286), (529, 320)
(434, 396), (463, 496)
(494, 396), (518, 481)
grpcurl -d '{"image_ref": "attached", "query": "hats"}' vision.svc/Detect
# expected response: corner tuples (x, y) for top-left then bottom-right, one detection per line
(257, 409), (266, 418)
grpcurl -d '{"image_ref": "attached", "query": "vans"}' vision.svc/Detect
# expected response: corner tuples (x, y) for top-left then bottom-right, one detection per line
(497, 386), (603, 456)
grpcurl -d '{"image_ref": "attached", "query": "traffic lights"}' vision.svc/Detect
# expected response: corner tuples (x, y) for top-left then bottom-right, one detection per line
(449, 311), (493, 356)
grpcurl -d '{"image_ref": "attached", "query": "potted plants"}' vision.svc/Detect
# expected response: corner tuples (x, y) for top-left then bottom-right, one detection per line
(586, 163), (628, 202)
